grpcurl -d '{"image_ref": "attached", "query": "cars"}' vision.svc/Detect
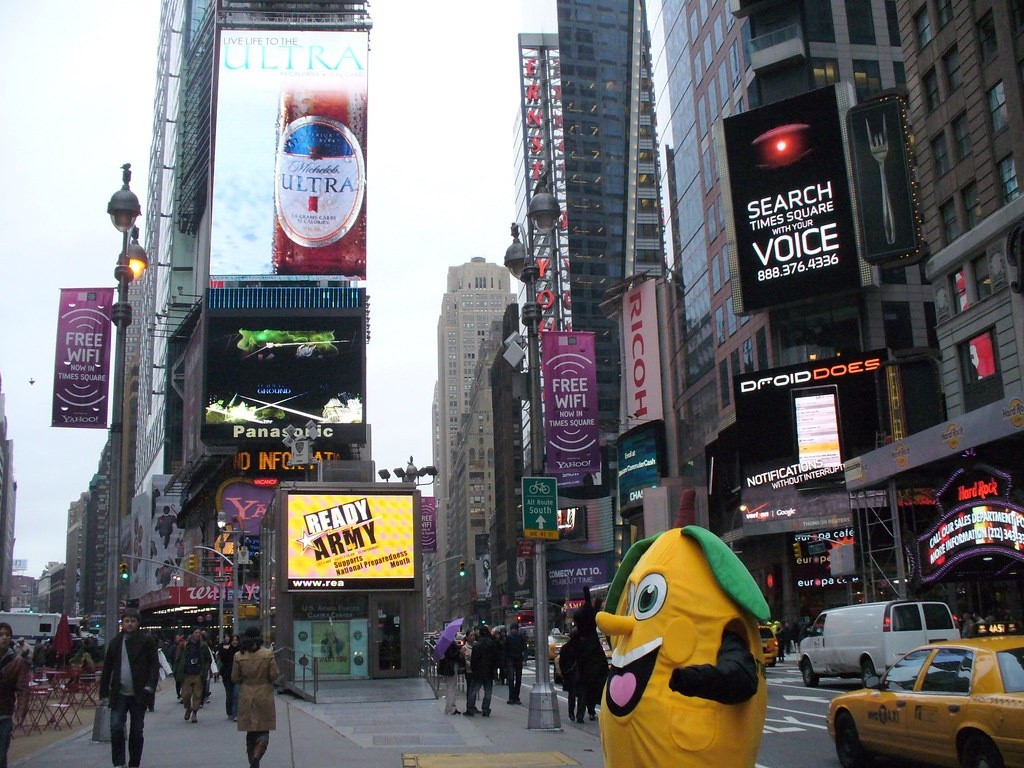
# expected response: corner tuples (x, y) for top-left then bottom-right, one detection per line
(518, 627), (535, 660)
(759, 626), (779, 666)
(548, 628), (570, 662)
(826, 633), (1024, 768)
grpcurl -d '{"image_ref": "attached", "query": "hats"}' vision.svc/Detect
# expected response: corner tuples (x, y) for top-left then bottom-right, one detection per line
(767, 621), (781, 625)
(17, 637), (24, 641)
(511, 622), (519, 628)
(190, 625), (200, 635)
(479, 626), (489, 636)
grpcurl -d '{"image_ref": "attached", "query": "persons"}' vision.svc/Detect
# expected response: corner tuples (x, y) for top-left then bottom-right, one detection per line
(444, 623), (528, 718)
(429, 636), (436, 657)
(321, 625), (345, 657)
(765, 620), (814, 663)
(171, 626), (241, 723)
(99, 608), (159, 768)
(134, 506), (184, 589)
(9, 633), (98, 693)
(559, 626), (596, 723)
(231, 626), (280, 768)
(0, 622), (29, 768)
(961, 610), (1013, 639)
(483, 560), (492, 597)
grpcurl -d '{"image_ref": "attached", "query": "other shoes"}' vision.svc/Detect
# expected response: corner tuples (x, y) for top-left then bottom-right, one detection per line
(473, 707), (482, 713)
(589, 714), (595, 720)
(576, 719), (585, 724)
(184, 707), (197, 723)
(482, 708), (491, 717)
(227, 714), (238, 721)
(463, 710), (474, 717)
(114, 764), (127, 768)
(177, 697), (183, 702)
(206, 699), (210, 703)
(449, 707), (461, 715)
(777, 659), (784, 663)
(507, 700), (521, 705)
(569, 715), (575, 721)
(200, 705), (203, 707)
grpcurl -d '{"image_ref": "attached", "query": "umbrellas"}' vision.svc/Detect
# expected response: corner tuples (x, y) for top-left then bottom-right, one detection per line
(434, 617), (464, 663)
(52, 610), (72, 671)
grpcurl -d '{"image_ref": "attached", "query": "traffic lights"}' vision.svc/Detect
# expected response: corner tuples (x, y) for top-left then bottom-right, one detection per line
(459, 561), (467, 577)
(563, 603), (567, 612)
(480, 619), (487, 625)
(793, 543), (802, 561)
(120, 564), (129, 580)
(513, 601), (519, 610)
(189, 554), (195, 570)
(223, 615), (234, 627)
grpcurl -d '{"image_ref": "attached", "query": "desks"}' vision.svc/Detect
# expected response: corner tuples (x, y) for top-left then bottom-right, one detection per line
(14, 689), (49, 732)
(43, 670), (67, 699)
(74, 677), (97, 704)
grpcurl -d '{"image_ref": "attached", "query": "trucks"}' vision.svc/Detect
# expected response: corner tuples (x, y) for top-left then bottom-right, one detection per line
(0, 608), (83, 641)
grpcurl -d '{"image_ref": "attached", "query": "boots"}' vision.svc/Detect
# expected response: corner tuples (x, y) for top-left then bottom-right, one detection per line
(246, 739), (268, 768)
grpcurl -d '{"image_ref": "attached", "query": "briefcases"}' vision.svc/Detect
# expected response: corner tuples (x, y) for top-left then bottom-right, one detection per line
(92, 706), (128, 742)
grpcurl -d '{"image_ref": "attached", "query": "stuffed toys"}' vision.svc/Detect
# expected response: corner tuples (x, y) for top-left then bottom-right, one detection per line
(595, 525), (770, 768)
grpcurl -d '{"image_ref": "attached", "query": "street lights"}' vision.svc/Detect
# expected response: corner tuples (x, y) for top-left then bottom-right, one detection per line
(90, 163), (148, 742)
(729, 505), (747, 553)
(503, 163), (572, 731)
(172, 575), (181, 606)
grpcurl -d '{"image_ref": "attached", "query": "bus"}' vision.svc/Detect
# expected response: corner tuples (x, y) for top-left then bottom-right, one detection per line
(516, 610), (534, 626)
(90, 614), (106, 626)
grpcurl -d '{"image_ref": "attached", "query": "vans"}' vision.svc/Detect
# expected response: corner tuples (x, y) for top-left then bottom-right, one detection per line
(797, 601), (961, 688)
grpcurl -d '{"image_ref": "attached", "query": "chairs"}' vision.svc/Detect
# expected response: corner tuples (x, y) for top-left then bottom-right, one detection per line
(11, 663), (104, 740)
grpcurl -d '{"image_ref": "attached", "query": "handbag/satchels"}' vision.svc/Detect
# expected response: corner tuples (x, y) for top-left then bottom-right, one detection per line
(438, 658), (456, 676)
